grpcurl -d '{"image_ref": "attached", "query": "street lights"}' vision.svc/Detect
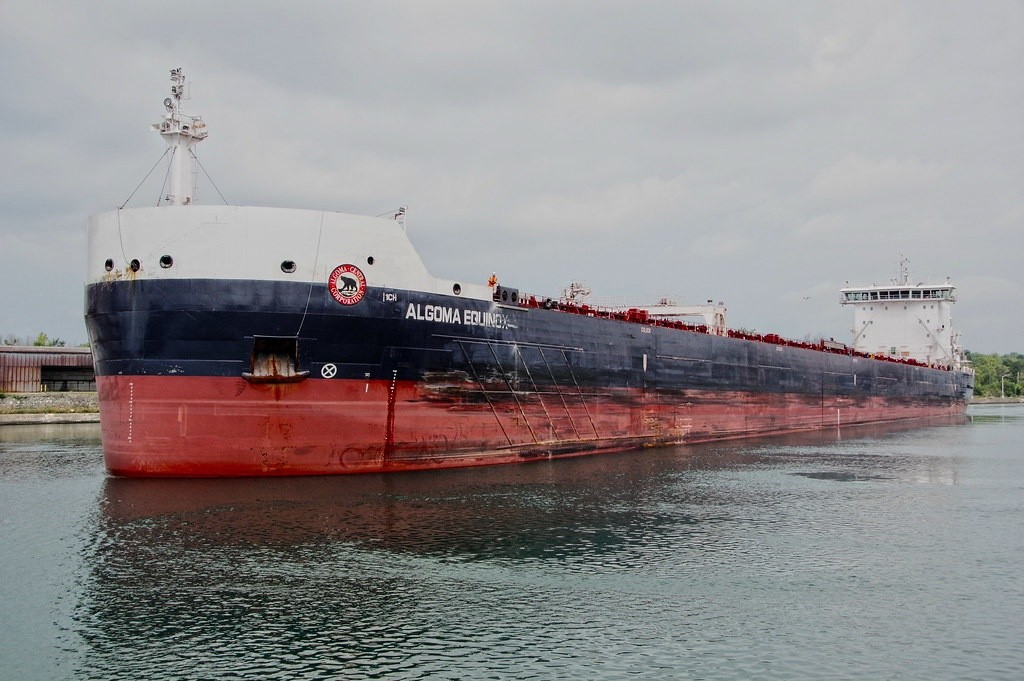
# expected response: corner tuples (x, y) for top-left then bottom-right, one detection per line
(1001, 374), (1013, 398)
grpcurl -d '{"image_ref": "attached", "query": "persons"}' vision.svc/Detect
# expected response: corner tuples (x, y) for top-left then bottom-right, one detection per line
(488, 272), (497, 288)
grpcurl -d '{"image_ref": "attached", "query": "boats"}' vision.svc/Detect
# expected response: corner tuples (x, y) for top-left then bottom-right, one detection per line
(83, 68), (977, 479)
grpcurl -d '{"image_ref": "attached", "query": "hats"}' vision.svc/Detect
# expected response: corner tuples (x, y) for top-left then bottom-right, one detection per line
(492, 272), (496, 275)
